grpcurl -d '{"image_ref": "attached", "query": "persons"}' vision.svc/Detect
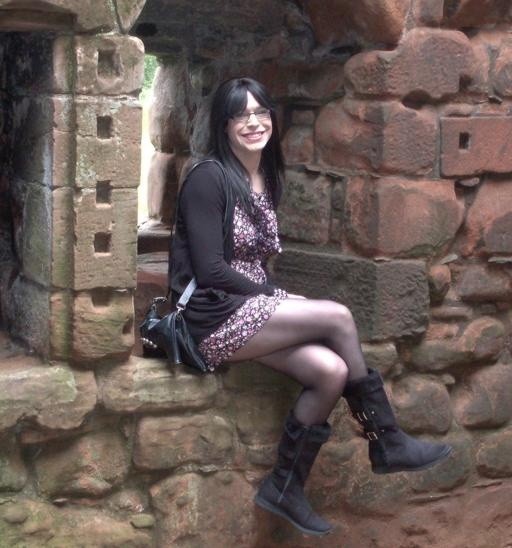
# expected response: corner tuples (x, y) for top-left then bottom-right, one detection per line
(168, 77), (454, 536)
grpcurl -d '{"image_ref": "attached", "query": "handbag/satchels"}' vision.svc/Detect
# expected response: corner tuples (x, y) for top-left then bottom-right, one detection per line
(140, 303), (207, 372)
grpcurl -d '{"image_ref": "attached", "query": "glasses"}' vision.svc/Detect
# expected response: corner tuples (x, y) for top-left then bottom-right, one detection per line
(228, 108), (271, 120)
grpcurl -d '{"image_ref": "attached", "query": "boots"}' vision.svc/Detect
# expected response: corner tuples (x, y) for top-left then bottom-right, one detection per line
(341, 367), (454, 474)
(253, 409), (332, 535)
